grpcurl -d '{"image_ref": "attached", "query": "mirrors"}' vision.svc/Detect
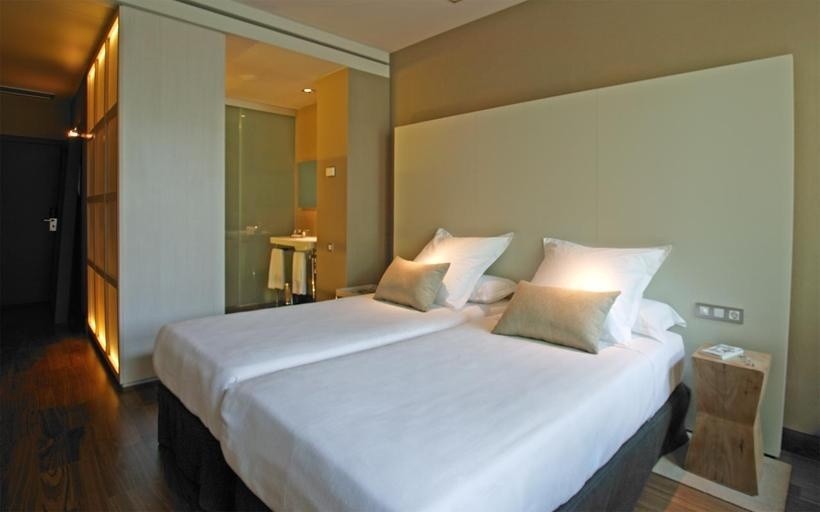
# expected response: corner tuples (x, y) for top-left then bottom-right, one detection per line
(297, 159), (316, 209)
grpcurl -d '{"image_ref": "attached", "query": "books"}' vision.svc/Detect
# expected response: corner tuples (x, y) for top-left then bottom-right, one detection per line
(702, 342), (743, 360)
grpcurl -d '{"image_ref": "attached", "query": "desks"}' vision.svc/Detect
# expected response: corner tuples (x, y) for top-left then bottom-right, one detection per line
(683, 340), (773, 497)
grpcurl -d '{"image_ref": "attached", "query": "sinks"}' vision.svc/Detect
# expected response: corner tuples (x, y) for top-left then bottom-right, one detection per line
(225, 230), (270, 240)
(269, 236), (317, 252)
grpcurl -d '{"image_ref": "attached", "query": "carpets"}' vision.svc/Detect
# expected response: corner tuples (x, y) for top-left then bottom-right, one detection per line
(651, 432), (793, 512)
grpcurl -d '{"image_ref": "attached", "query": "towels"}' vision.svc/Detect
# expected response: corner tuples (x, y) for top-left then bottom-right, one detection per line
(268, 248), (307, 295)
